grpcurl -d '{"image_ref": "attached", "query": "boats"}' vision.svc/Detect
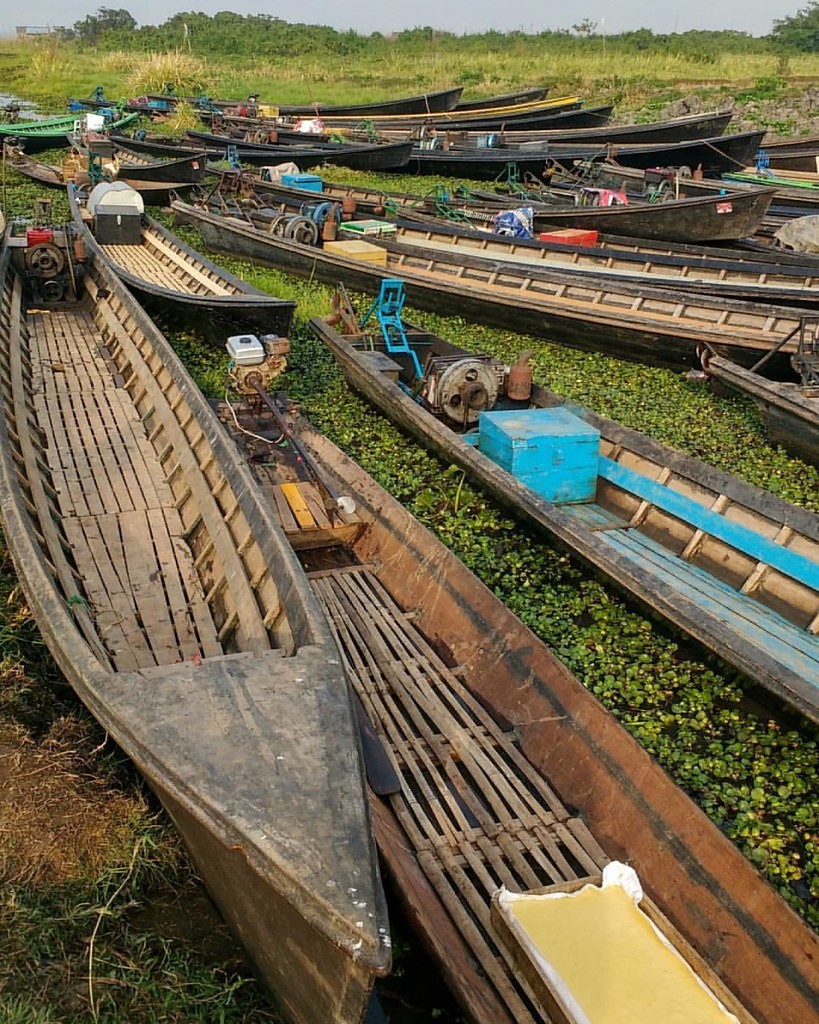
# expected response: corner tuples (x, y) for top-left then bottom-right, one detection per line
(425, 177), (777, 246)
(2, 195), (394, 1024)
(207, 146), (807, 306)
(66, 94), (166, 113)
(700, 321), (818, 468)
(173, 191), (818, 379)
(3, 136), (196, 205)
(74, 88), (216, 116)
(308, 275), (818, 725)
(0, 95), (137, 154)
(77, 82), (819, 237)
(204, 333), (819, 1024)
(66, 119), (207, 183)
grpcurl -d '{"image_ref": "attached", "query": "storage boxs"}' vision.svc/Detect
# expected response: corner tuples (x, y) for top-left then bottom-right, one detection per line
(519, 140), (549, 152)
(479, 408), (602, 507)
(147, 101), (167, 108)
(282, 173), (323, 192)
(340, 219), (396, 242)
(88, 140), (112, 159)
(540, 228), (598, 247)
(93, 205), (141, 245)
(323, 240), (387, 266)
(258, 104), (280, 118)
